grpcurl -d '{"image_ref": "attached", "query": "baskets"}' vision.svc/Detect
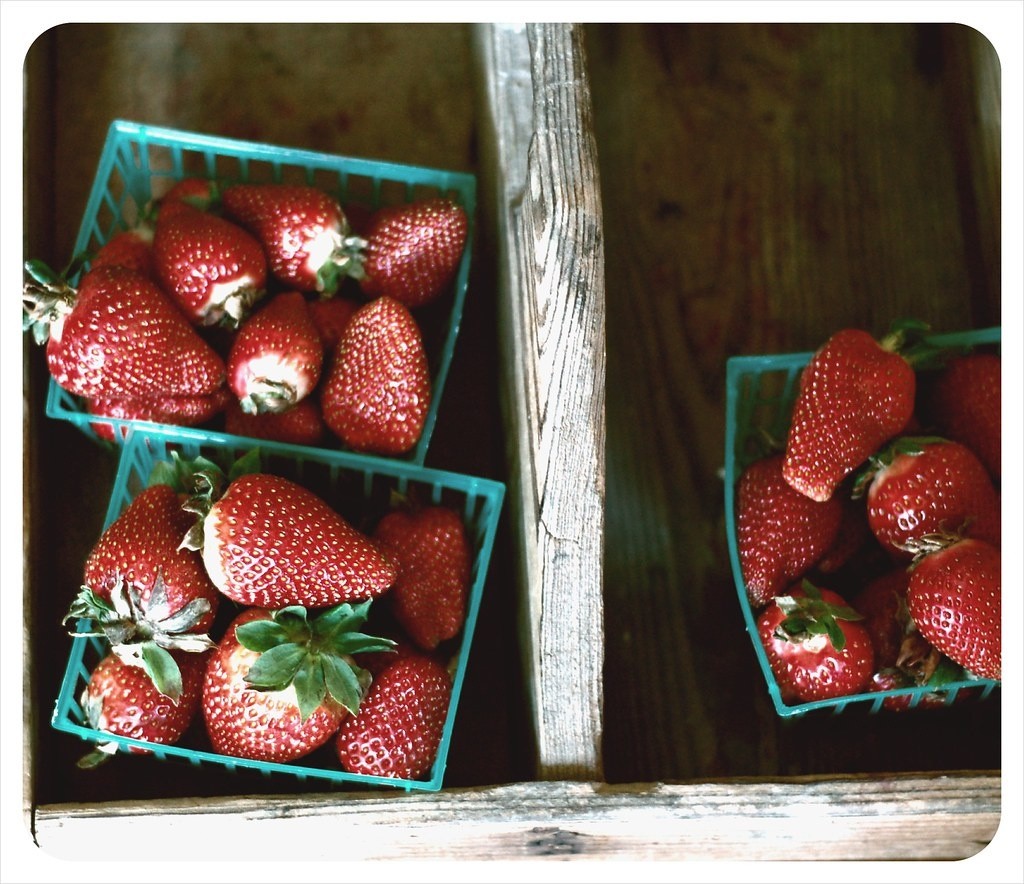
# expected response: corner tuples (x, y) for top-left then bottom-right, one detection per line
(51, 422), (508, 795)
(720, 323), (1001, 726)
(44, 118), (474, 464)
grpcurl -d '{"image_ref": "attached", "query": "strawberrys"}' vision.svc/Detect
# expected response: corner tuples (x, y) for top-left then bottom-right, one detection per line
(733, 320), (1002, 709)
(61, 449), (475, 783)
(23, 173), (466, 458)
